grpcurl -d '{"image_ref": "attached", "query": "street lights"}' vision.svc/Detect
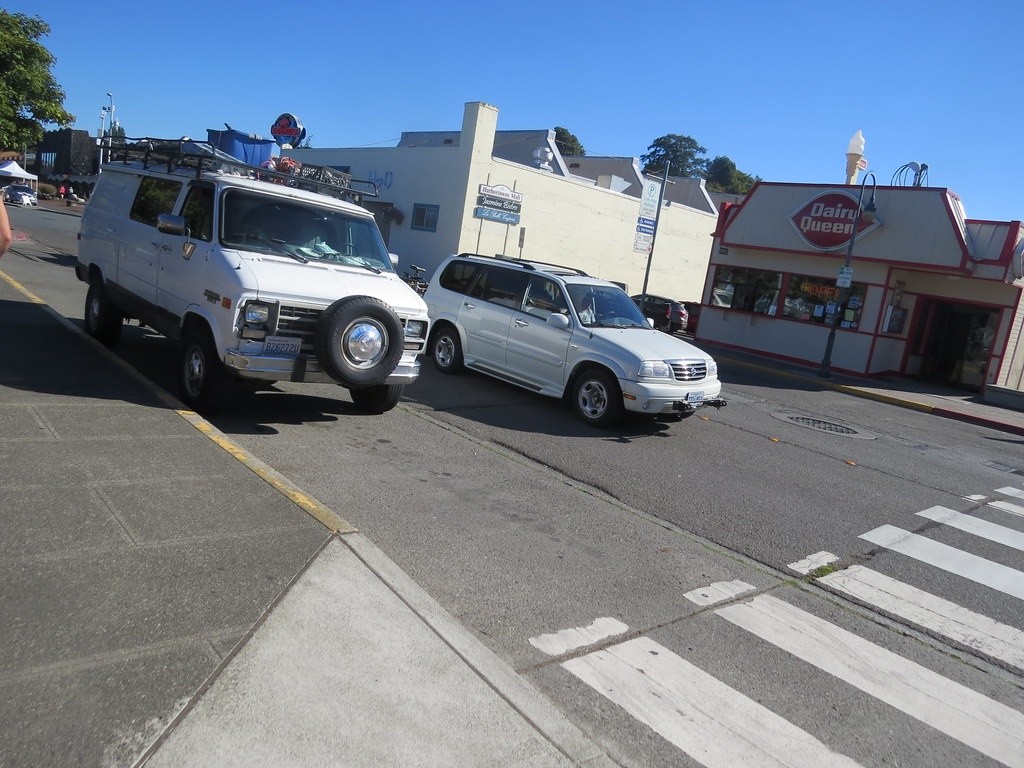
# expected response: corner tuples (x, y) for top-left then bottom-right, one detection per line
(99, 92), (120, 175)
(816, 171), (877, 380)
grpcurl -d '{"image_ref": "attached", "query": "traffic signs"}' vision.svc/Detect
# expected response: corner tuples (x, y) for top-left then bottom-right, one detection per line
(633, 174), (664, 255)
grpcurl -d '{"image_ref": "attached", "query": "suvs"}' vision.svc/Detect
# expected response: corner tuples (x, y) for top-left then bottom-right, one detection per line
(75, 136), (431, 414)
(422, 252), (727, 428)
(606, 281), (701, 333)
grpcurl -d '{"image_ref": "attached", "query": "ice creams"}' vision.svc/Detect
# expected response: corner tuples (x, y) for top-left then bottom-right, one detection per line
(845, 129), (865, 185)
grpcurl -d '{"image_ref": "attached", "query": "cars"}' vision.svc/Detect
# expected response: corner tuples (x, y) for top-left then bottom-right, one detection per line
(3, 185), (38, 206)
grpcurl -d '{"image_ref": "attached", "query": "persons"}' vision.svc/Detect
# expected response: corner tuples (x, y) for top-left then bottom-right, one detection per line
(68, 186), (73, 195)
(59, 186), (66, 199)
(0, 195), (12, 257)
(575, 290), (596, 324)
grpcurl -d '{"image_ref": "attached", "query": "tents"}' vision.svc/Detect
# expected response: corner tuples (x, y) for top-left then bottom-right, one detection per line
(0, 160), (38, 192)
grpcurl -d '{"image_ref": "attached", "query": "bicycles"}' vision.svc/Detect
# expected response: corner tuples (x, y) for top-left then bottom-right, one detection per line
(403, 265), (429, 295)
